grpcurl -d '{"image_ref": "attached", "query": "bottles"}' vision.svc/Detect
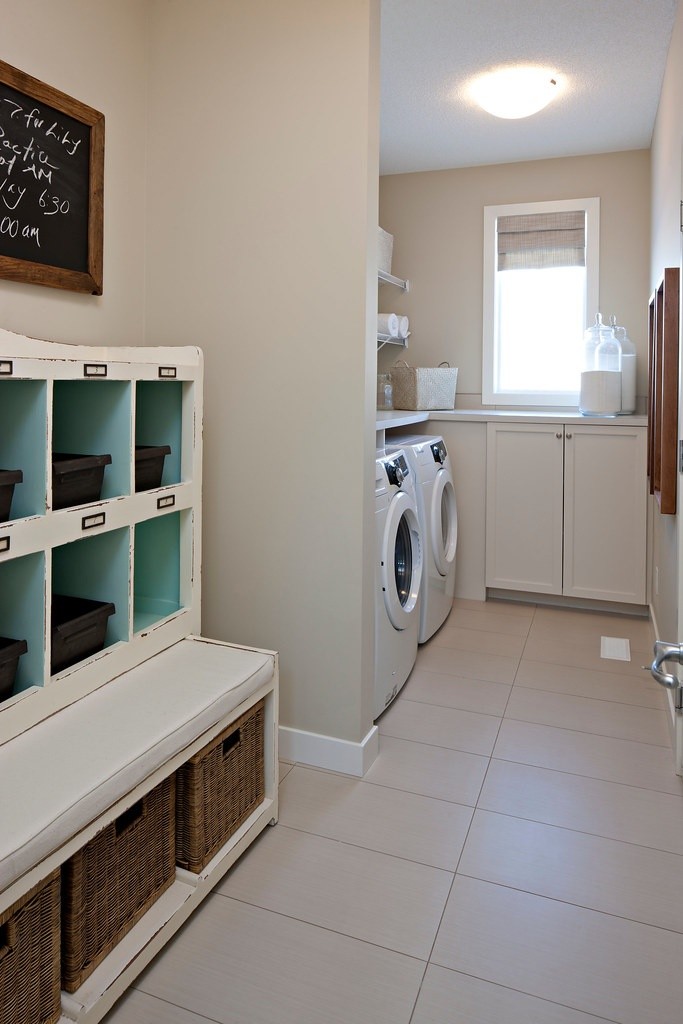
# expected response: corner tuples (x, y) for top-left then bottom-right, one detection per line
(378, 375), (393, 410)
(578, 313), (622, 418)
(596, 315), (636, 414)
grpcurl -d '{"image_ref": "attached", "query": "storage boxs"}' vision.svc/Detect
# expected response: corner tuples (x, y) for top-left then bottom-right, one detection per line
(0, 637), (28, 704)
(135, 445), (171, 493)
(51, 593), (116, 676)
(0, 469), (23, 524)
(52, 452), (112, 511)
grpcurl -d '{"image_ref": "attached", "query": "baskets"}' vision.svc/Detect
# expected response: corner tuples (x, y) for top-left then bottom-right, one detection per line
(1, 691), (266, 1023)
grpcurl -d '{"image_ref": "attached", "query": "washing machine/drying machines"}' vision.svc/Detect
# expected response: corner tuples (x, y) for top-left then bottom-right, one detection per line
(369, 447), (423, 722)
(384, 427), (459, 648)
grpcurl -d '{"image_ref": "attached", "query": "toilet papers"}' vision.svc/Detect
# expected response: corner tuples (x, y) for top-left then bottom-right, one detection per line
(377, 312), (411, 338)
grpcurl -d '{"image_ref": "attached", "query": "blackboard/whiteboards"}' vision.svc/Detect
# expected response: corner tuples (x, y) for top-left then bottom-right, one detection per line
(0, 59), (105, 295)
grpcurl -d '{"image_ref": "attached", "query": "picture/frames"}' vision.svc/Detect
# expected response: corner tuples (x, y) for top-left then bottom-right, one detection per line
(647, 267), (680, 514)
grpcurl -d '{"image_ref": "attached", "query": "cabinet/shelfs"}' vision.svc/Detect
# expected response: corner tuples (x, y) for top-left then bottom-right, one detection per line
(485, 422), (650, 619)
(378, 270), (410, 347)
(1, 329), (280, 1024)
